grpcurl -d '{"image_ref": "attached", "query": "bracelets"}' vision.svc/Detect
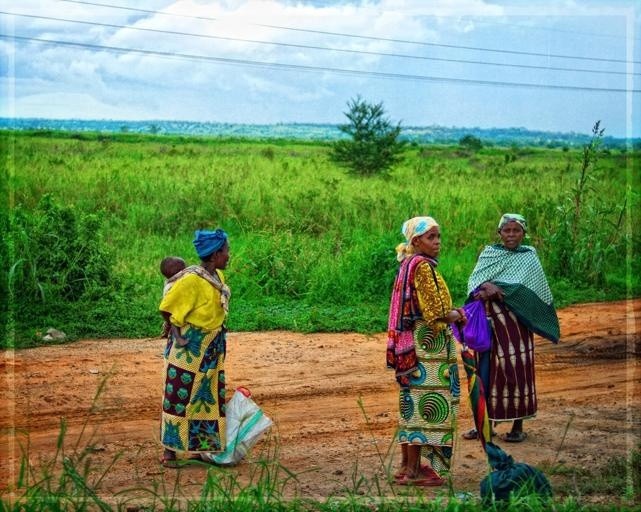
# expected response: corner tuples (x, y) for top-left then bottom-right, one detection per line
(454, 306), (467, 322)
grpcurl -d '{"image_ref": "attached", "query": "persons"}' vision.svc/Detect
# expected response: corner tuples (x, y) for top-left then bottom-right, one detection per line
(386, 217), (470, 487)
(460, 212), (560, 442)
(158, 255), (194, 347)
(158, 227), (232, 471)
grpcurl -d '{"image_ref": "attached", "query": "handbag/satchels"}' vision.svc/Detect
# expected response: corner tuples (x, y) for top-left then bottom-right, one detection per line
(202, 389), (273, 467)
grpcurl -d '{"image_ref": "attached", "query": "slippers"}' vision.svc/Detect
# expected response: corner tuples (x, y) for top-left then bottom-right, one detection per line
(504, 431), (526, 442)
(190, 455), (201, 460)
(394, 463), (443, 486)
(157, 457), (178, 467)
(463, 426), (497, 438)
